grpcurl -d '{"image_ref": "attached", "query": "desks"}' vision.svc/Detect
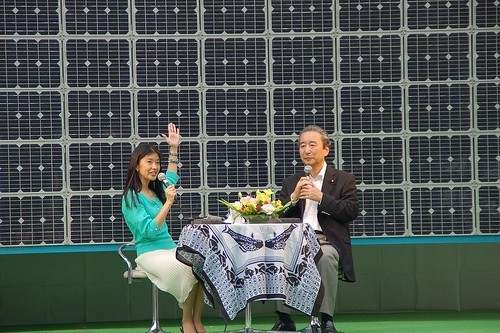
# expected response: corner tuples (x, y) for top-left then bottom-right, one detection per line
(175, 222), (323, 333)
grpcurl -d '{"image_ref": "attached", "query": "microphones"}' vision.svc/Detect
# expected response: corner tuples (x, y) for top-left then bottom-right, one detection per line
(157, 173), (178, 200)
(303, 165), (312, 187)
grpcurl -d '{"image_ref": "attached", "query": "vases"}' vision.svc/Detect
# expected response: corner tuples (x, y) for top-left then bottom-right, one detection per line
(245, 213), (271, 223)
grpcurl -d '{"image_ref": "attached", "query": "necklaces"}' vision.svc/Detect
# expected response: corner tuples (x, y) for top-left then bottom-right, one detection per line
(143, 186), (150, 194)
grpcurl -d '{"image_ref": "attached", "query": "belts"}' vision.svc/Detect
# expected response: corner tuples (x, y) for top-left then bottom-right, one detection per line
(314, 230), (324, 234)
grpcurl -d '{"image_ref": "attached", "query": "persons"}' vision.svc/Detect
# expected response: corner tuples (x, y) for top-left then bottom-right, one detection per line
(121, 123), (208, 333)
(271, 125), (359, 333)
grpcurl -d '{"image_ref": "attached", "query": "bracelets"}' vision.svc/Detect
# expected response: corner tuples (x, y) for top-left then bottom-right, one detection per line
(169, 152), (177, 156)
(168, 158), (178, 163)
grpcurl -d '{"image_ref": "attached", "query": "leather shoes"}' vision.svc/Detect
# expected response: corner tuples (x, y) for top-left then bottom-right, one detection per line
(271, 319), (296, 331)
(320, 322), (337, 333)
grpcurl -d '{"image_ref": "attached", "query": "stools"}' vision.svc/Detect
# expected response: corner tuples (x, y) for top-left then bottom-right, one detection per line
(118, 241), (170, 333)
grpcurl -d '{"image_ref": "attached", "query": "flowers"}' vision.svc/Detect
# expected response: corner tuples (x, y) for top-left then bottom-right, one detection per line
(219, 187), (296, 217)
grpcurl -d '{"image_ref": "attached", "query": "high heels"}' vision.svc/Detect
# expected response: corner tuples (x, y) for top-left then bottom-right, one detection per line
(180, 318), (184, 333)
